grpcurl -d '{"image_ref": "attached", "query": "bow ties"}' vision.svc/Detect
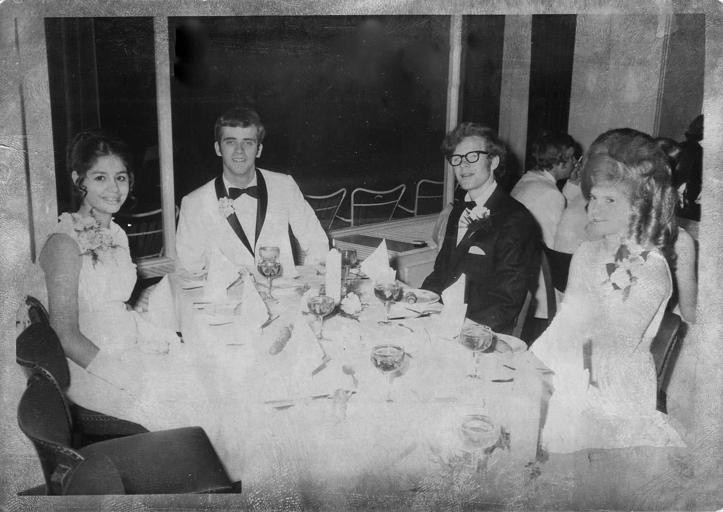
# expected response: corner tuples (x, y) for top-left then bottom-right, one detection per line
(229, 186), (258, 200)
(457, 200), (476, 210)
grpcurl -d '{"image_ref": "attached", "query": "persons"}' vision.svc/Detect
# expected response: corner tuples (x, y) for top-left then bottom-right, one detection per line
(175, 103), (333, 284)
(32, 128), (184, 430)
(507, 113), (708, 325)
(526, 151), (675, 413)
(408, 119), (540, 340)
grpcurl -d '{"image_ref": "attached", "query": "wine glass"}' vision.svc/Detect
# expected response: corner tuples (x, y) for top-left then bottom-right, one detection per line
(257, 245), (282, 300)
(336, 244), (357, 297)
(373, 282), (401, 328)
(306, 282), (335, 342)
(457, 322), (490, 385)
(369, 340), (407, 408)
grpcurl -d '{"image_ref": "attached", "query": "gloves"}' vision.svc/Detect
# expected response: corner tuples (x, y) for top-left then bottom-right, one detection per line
(85, 348), (177, 405)
(130, 311), (170, 357)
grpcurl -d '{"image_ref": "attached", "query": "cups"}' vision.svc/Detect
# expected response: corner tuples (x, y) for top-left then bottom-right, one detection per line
(455, 395), (503, 449)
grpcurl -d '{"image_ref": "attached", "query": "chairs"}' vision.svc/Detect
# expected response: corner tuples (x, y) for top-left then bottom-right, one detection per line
(305, 178), (444, 233)
(117, 204), (179, 265)
(541, 243), (571, 323)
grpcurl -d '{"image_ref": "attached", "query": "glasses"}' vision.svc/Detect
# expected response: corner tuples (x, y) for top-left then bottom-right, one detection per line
(447, 151), (488, 166)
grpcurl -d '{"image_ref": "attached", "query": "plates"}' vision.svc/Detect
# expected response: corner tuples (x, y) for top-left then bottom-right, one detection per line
(399, 288), (441, 309)
(485, 332), (528, 356)
